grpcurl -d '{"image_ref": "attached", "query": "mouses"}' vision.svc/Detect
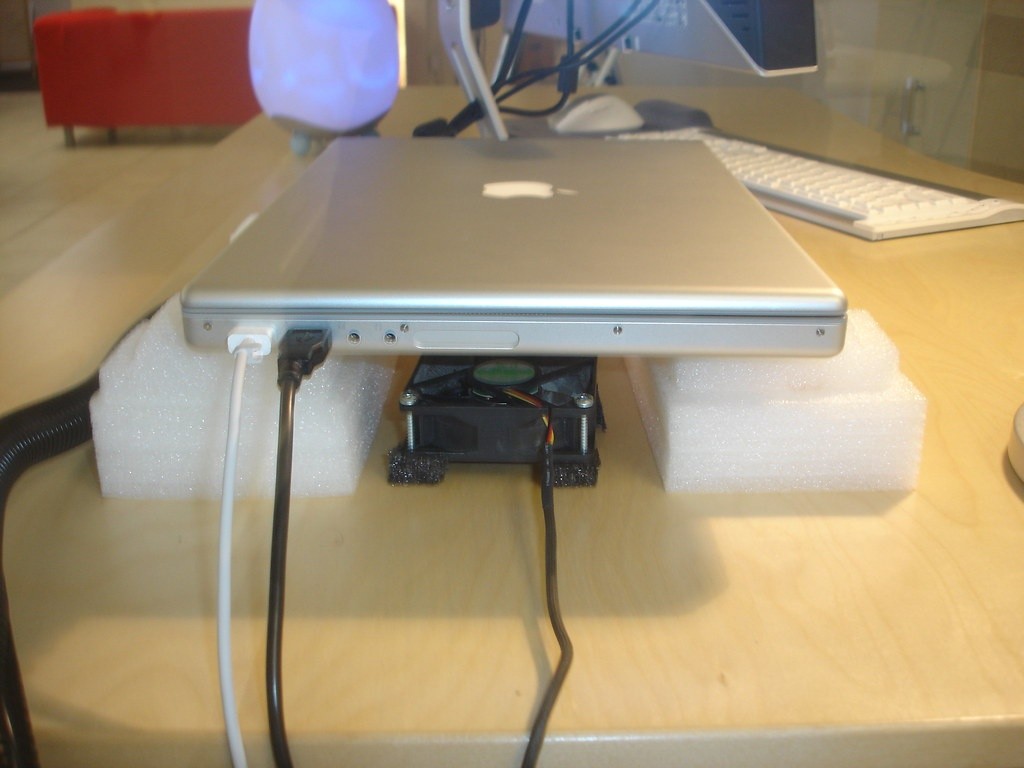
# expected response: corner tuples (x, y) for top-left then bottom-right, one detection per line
(548, 91), (644, 135)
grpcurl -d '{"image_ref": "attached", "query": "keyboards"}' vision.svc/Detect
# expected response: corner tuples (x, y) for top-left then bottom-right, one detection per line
(606, 128), (1024, 242)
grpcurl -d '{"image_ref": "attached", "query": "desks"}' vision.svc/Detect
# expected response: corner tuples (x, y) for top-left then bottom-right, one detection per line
(1, 85), (1024, 768)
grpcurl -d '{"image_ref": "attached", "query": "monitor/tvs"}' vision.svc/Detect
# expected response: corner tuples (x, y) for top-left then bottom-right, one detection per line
(441, 0), (819, 140)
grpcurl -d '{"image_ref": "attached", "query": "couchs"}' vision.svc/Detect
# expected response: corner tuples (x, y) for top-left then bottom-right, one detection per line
(33, 0), (262, 145)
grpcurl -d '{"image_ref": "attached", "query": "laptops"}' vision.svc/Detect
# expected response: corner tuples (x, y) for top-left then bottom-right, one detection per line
(181, 137), (848, 359)
